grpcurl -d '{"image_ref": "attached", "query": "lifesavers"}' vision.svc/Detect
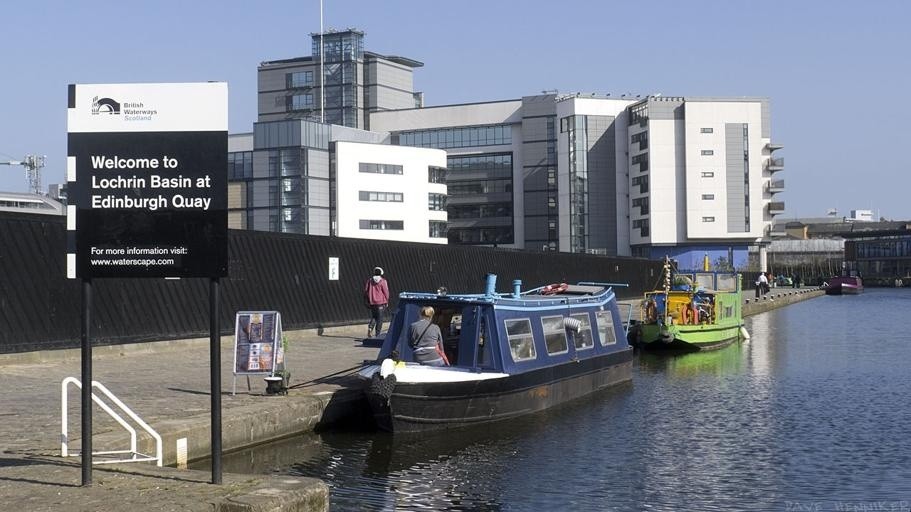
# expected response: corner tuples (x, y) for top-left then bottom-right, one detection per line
(542, 283), (568, 294)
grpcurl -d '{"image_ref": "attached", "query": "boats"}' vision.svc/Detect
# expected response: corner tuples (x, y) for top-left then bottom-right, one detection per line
(656, 339), (746, 376)
(631, 250), (747, 352)
(825, 273), (863, 295)
(355, 275), (638, 436)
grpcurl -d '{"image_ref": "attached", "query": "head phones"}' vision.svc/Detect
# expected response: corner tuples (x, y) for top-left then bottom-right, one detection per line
(373, 266), (384, 275)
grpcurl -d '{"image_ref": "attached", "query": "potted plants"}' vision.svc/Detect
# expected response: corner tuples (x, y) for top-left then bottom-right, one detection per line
(272, 337), (291, 387)
(263, 377), (285, 396)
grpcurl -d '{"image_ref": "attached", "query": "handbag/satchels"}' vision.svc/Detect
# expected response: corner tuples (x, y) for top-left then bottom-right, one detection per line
(364, 291), (374, 309)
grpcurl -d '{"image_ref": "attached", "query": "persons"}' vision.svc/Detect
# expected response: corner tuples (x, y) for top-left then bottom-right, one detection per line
(362, 265), (390, 339)
(407, 305), (451, 367)
(769, 272), (802, 289)
(756, 272), (769, 296)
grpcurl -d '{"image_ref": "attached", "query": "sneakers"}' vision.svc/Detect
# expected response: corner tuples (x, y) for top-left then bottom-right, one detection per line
(368, 328), (372, 338)
